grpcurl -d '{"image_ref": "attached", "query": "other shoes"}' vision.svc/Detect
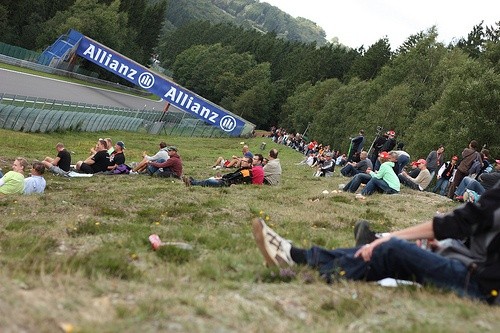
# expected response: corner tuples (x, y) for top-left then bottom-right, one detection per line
(355, 194), (365, 199)
(183, 176), (190, 186)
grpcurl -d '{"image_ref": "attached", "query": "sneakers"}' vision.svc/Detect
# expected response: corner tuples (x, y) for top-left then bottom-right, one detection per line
(252, 217), (297, 273)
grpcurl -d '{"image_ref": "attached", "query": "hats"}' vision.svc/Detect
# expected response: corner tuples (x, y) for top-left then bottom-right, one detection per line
(453, 156), (458, 161)
(117, 141), (126, 150)
(378, 152), (389, 158)
(168, 146), (177, 152)
(326, 152), (333, 157)
(410, 162), (418, 167)
(416, 159), (426, 164)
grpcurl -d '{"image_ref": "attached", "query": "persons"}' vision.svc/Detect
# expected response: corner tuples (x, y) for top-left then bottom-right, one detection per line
(128, 142), (183, 178)
(253, 189), (500, 303)
(370, 130), (396, 171)
(40, 142), (71, 172)
(266, 126), (345, 177)
(340, 141), (500, 202)
(347, 130), (365, 163)
(354, 220), (389, 249)
(0, 157), (46, 196)
(183, 145), (282, 186)
(73, 138), (126, 174)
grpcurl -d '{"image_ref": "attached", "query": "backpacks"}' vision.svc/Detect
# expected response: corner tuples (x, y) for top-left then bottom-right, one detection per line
(469, 152), (481, 174)
